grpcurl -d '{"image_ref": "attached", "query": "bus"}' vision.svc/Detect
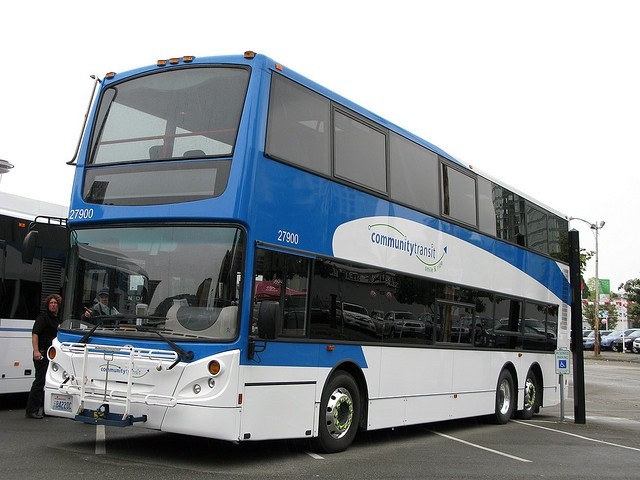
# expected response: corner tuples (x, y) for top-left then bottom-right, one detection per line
(0, 191), (69, 395)
(43, 50), (572, 452)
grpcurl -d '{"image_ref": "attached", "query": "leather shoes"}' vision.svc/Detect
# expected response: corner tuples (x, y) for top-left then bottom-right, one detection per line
(25, 412), (43, 419)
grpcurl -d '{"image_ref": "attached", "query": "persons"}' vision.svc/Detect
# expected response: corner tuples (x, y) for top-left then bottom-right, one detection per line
(81, 288), (119, 325)
(24, 294), (63, 419)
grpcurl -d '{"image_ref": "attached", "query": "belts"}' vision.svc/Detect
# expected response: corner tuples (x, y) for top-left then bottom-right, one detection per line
(40, 349), (45, 352)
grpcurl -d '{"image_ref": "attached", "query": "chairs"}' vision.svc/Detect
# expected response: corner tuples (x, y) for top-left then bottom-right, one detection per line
(149, 145), (164, 159)
(183, 150), (206, 158)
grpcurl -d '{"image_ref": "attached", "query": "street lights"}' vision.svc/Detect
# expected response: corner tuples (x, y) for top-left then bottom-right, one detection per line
(568, 217), (605, 356)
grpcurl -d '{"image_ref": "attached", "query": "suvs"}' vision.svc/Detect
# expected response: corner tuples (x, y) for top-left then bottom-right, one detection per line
(612, 329), (640, 353)
(500, 319), (556, 339)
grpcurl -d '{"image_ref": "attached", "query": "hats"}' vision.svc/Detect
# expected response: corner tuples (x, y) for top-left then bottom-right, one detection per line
(98, 287), (109, 295)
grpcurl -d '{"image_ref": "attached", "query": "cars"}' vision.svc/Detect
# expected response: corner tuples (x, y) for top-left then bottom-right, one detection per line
(633, 337), (640, 352)
(336, 301), (377, 333)
(482, 323), (557, 351)
(422, 312), (470, 340)
(383, 310), (426, 339)
(600, 330), (624, 351)
(370, 310), (384, 329)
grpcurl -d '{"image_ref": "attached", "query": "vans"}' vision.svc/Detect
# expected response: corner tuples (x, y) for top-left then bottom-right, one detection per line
(583, 331), (613, 348)
